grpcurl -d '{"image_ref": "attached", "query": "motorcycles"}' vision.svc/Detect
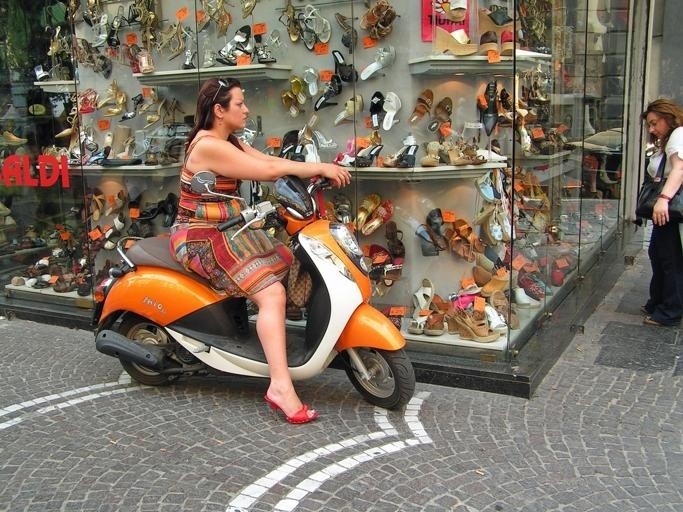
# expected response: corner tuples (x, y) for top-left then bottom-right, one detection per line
(91, 170), (416, 411)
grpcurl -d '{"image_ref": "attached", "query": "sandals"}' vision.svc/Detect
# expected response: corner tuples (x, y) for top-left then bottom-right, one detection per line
(330, 165), (608, 342)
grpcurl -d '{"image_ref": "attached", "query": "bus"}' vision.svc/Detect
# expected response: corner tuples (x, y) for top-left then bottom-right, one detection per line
(599, 98), (630, 131)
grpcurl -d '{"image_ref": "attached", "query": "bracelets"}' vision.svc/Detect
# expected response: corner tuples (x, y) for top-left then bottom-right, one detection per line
(656, 193), (672, 203)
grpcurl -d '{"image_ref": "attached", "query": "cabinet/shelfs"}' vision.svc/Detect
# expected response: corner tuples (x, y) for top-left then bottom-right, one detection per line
(34, 56), (577, 352)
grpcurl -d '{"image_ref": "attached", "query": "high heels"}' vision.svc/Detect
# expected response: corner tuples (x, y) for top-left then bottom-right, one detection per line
(264, 396), (317, 426)
(0, 0), (197, 176)
(198, 0), (576, 168)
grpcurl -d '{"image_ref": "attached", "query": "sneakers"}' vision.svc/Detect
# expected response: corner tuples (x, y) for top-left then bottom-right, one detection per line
(639, 305), (660, 326)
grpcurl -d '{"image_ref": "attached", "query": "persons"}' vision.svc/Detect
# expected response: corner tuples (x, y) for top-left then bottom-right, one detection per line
(168, 72), (353, 425)
(636, 97), (682, 332)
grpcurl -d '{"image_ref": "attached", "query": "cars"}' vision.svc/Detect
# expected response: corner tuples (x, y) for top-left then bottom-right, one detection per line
(563, 127), (623, 199)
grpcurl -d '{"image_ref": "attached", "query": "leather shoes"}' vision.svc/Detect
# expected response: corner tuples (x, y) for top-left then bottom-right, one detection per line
(288, 304), (303, 321)
(0, 189), (179, 296)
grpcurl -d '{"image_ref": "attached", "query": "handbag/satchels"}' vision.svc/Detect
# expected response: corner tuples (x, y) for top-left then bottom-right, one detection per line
(285, 238), (314, 310)
(635, 178), (683, 223)
